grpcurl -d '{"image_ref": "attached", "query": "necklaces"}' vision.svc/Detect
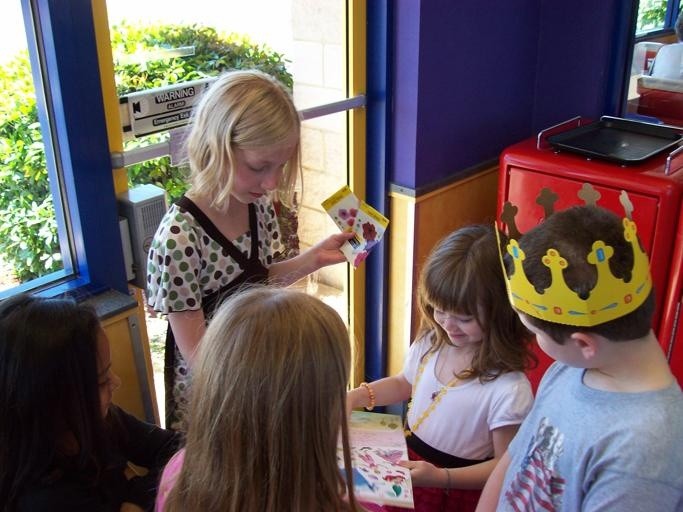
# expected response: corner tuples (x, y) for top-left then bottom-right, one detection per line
(402, 350), (470, 439)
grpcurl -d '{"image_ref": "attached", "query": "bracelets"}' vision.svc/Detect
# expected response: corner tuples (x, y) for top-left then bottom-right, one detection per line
(359, 381), (375, 411)
(442, 468), (452, 497)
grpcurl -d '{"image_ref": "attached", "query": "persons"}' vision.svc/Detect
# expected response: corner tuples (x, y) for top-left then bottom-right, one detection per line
(341, 223), (534, 512)
(145, 68), (357, 432)
(0, 290), (185, 512)
(647, 6), (683, 82)
(152, 280), (374, 512)
(470, 203), (682, 511)
